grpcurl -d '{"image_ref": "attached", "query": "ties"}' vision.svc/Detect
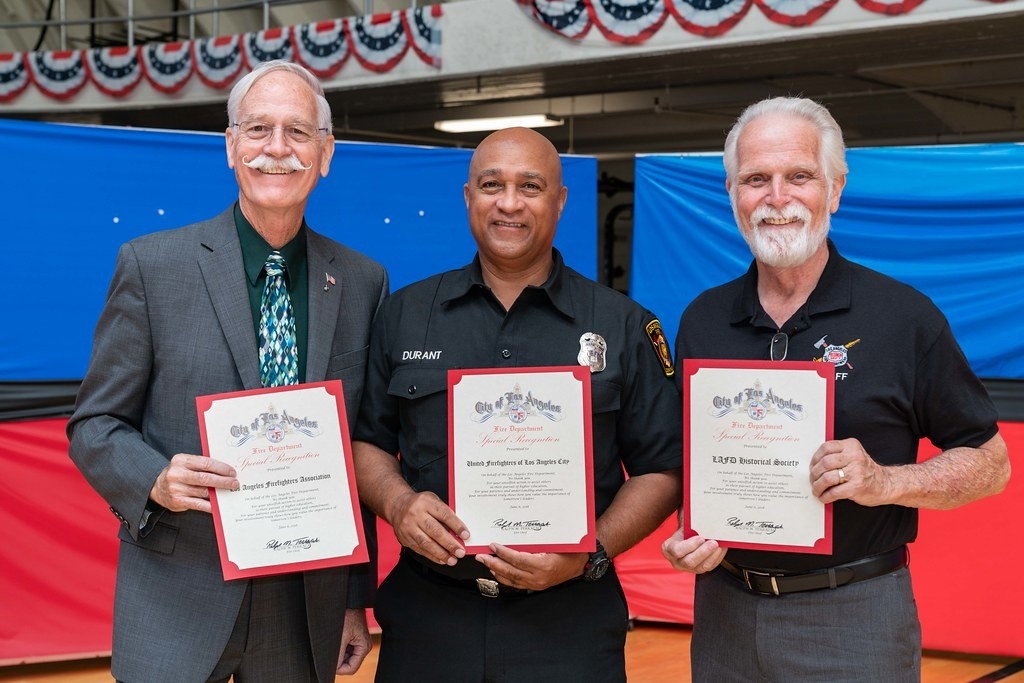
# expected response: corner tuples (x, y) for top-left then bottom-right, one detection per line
(259, 251), (298, 388)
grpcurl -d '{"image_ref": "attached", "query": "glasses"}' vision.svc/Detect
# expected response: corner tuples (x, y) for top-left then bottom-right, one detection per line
(231, 120), (329, 145)
(770, 329), (788, 360)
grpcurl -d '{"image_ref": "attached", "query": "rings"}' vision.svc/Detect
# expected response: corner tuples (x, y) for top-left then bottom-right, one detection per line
(838, 468), (845, 483)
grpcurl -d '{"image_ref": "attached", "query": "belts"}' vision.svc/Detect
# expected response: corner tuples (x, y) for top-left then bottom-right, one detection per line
(402, 553), (529, 599)
(719, 545), (908, 596)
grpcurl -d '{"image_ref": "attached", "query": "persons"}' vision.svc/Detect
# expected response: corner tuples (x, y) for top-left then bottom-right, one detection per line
(661, 95), (1012, 683)
(66, 59), (390, 683)
(350, 127), (683, 683)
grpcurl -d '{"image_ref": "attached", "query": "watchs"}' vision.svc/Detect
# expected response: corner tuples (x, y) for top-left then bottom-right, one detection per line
(582, 538), (610, 583)
